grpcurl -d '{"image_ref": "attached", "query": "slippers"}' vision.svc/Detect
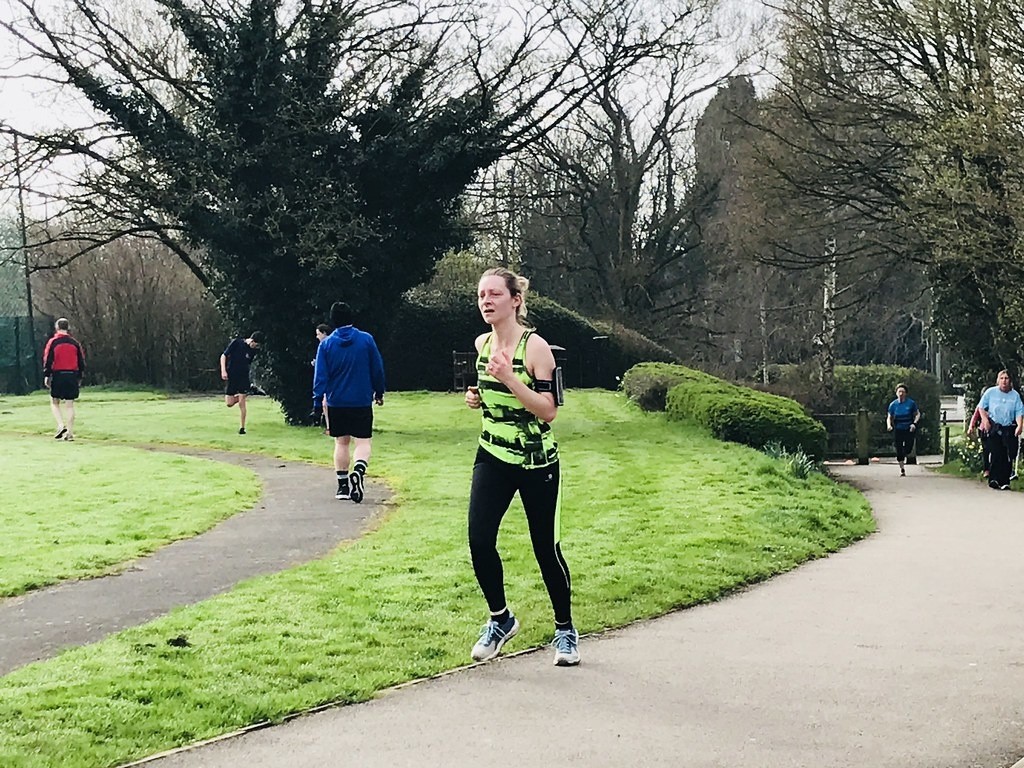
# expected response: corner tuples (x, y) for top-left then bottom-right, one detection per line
(54, 428), (67, 439)
(64, 437), (74, 441)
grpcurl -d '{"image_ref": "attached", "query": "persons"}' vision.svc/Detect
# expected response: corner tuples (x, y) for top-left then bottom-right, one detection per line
(311, 303), (385, 503)
(44, 317), (85, 441)
(220, 331), (264, 436)
(466, 268), (580, 666)
(887, 383), (920, 476)
(967, 370), (1024, 490)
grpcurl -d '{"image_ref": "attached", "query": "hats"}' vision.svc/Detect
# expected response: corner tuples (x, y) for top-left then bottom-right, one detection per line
(329, 302), (352, 325)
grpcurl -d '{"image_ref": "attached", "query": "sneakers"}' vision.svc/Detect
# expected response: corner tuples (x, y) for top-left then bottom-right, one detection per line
(549, 629), (580, 666)
(238, 427), (246, 435)
(988, 480), (999, 490)
(471, 615), (520, 662)
(901, 470), (906, 477)
(250, 386), (266, 396)
(983, 471), (990, 477)
(334, 486), (350, 500)
(1001, 485), (1011, 489)
(350, 471), (364, 503)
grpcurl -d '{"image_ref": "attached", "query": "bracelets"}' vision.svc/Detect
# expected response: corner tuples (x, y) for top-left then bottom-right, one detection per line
(913, 423), (917, 426)
